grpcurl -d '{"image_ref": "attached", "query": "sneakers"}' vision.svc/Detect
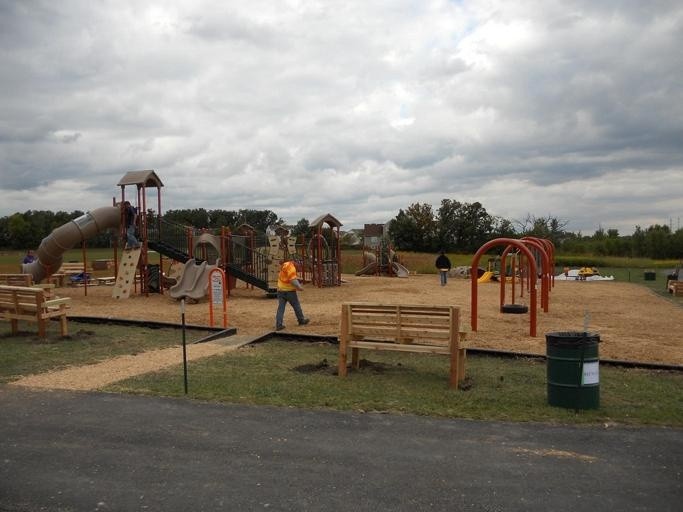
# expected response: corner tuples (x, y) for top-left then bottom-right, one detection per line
(276, 325), (285, 331)
(298, 318), (309, 326)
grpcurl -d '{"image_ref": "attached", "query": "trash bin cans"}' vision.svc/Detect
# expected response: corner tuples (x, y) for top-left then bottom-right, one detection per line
(667, 274), (678, 285)
(543, 332), (600, 410)
(644, 272), (656, 281)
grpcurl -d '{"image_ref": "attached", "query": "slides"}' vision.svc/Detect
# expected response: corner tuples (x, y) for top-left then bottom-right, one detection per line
(19, 207), (121, 280)
(477, 271), (494, 283)
(355, 251), (377, 276)
(169, 257), (222, 299)
(389, 251), (409, 278)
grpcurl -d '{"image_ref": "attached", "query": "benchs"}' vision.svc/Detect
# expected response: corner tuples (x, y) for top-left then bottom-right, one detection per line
(336, 300), (472, 391)
(0, 272), (72, 341)
(61, 263), (93, 281)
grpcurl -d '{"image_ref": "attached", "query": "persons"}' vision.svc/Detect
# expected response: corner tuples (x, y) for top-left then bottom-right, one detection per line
(22, 247), (37, 265)
(274, 254), (309, 332)
(666, 269), (679, 293)
(562, 264), (570, 276)
(433, 249), (451, 287)
(122, 199), (140, 250)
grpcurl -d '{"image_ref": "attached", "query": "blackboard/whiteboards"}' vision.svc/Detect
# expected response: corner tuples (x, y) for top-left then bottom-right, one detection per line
(147, 264), (160, 294)
